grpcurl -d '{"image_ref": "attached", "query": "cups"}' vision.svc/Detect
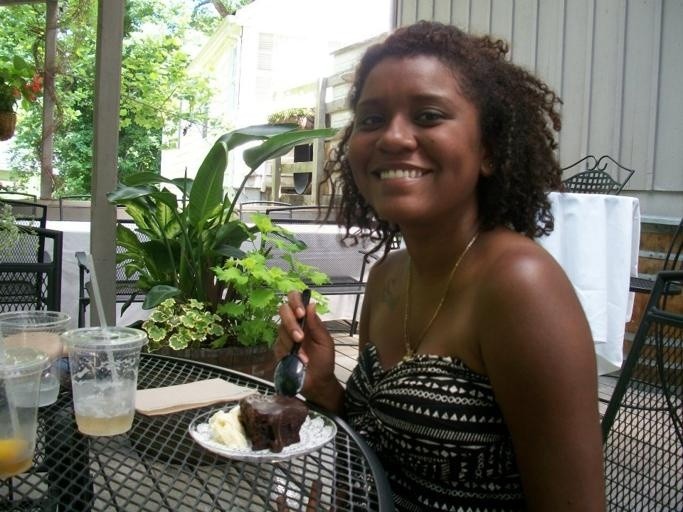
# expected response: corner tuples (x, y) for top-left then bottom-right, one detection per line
(1, 310), (72, 409)
(63, 326), (149, 437)
(0, 346), (52, 479)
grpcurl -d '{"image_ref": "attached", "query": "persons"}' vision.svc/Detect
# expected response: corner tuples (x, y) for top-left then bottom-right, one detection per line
(272, 20), (608, 507)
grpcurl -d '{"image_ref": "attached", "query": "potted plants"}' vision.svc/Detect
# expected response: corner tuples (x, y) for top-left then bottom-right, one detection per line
(106, 124), (344, 461)
(0, 56), (39, 140)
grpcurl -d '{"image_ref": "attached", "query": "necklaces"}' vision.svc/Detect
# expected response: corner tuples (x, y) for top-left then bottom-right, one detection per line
(404, 231), (481, 361)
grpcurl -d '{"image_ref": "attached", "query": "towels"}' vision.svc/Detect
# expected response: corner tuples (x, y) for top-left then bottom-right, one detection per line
(132, 376), (256, 417)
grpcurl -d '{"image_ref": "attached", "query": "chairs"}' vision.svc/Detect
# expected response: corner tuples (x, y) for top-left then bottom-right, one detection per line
(1, 200), (47, 299)
(558, 155), (635, 196)
(257, 205), (400, 347)
(596, 215), (682, 446)
(0, 192), (37, 217)
(0, 224), (63, 312)
(74, 219), (203, 343)
(239, 201), (292, 224)
(59, 195), (92, 222)
(599, 272), (683, 510)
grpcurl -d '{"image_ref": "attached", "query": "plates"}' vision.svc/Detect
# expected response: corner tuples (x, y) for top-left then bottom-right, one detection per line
(189, 406), (338, 461)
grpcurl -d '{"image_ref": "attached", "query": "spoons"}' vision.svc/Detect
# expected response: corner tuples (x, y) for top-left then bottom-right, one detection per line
(274, 288), (311, 399)
